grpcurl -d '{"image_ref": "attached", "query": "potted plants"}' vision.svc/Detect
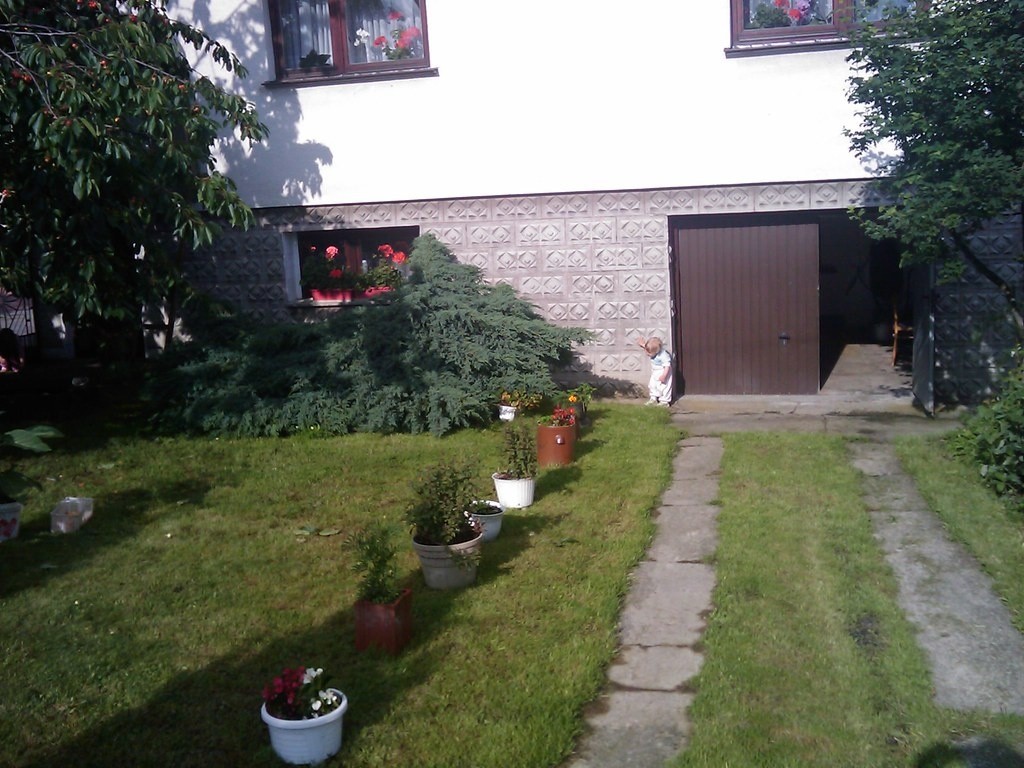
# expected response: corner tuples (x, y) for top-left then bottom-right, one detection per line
(463, 460), (505, 544)
(340, 514), (414, 658)
(402, 458), (487, 592)
(299, 49), (331, 69)
(492, 388), (542, 422)
(0, 411), (82, 545)
(492, 408), (537, 510)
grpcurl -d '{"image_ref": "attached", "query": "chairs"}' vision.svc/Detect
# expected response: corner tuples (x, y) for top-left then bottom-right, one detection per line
(891, 292), (914, 367)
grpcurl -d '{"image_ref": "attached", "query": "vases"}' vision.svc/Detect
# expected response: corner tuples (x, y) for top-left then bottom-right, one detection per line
(308, 287), (355, 301)
(537, 421), (576, 469)
(261, 688), (348, 765)
(569, 402), (586, 419)
(364, 286), (396, 299)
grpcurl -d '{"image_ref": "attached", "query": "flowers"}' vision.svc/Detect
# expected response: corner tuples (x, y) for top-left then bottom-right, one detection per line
(261, 663), (343, 721)
(744, 0), (801, 30)
(354, 243), (406, 294)
(298, 246), (360, 292)
(551, 381), (598, 413)
(537, 403), (577, 427)
(372, 10), (422, 61)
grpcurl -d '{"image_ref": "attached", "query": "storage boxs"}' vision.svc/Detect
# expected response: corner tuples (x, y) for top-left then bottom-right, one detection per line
(50, 496), (95, 535)
